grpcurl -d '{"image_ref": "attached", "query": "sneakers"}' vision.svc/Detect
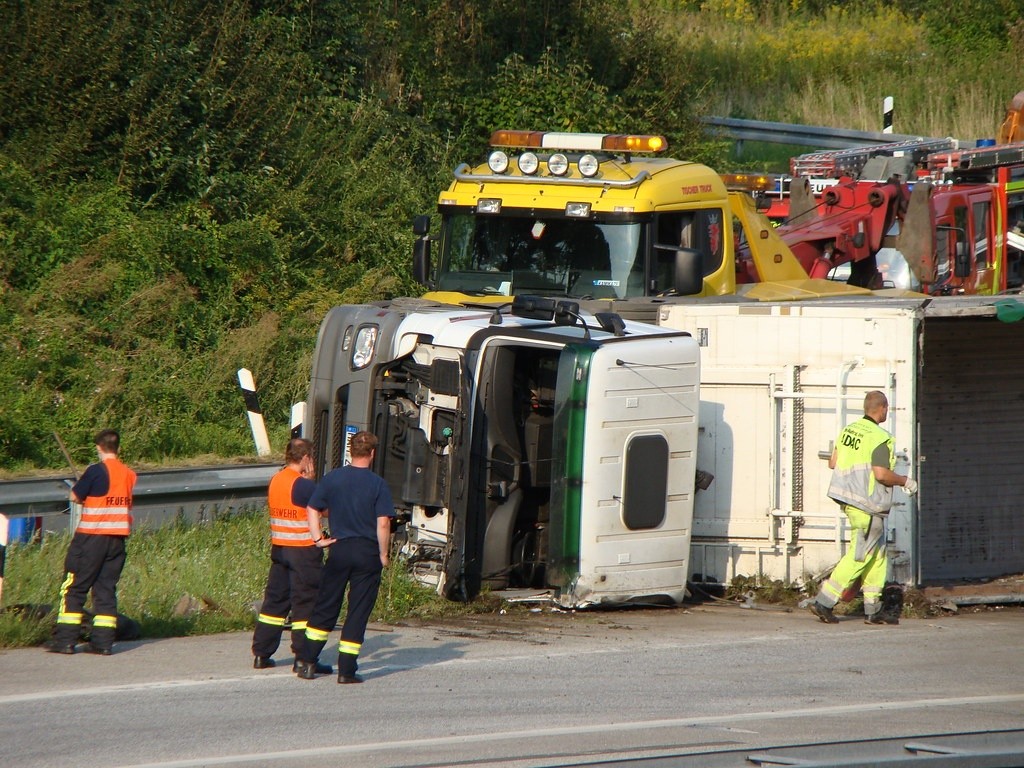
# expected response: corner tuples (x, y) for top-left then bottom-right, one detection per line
(866, 610), (900, 626)
(811, 602), (839, 623)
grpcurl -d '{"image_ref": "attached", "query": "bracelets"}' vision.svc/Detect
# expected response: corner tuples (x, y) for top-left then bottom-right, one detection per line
(313, 536), (322, 543)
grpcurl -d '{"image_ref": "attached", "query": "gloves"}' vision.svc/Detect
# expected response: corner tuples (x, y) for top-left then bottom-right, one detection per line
(900, 476), (919, 496)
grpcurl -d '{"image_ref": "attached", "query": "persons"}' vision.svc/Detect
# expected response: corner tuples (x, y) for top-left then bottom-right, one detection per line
(49, 430), (137, 655)
(808, 391), (918, 625)
(251, 438), (334, 675)
(297, 432), (397, 684)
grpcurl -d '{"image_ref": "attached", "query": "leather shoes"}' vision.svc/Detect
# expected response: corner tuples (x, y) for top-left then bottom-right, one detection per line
(297, 662), (316, 679)
(254, 655), (275, 668)
(294, 658), (333, 673)
(338, 673), (363, 683)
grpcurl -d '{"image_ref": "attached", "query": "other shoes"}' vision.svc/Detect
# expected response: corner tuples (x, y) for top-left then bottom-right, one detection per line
(84, 644), (112, 655)
(49, 644), (74, 654)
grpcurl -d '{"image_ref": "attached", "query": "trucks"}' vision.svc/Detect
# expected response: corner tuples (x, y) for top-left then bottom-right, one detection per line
(306, 286), (1024, 609)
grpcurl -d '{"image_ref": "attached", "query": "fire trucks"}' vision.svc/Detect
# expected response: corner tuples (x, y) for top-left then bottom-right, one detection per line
(412, 90), (1024, 302)
